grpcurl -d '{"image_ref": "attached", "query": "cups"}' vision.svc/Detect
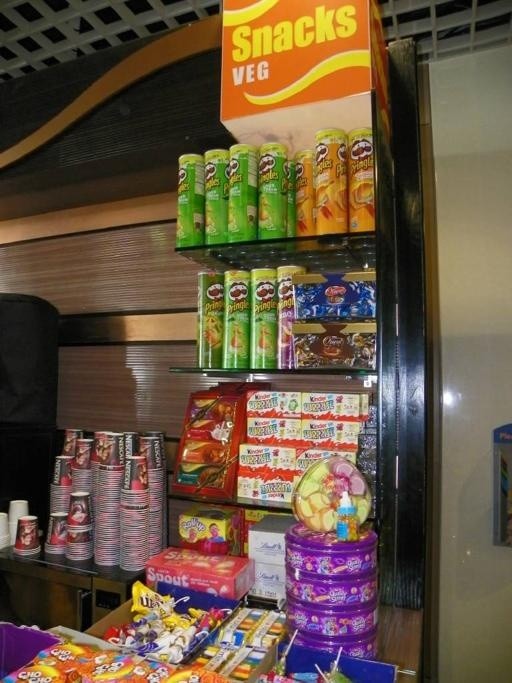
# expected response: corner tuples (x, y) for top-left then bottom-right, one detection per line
(0, 430), (165, 571)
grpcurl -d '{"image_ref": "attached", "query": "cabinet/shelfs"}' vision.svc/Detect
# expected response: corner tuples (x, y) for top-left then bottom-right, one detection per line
(165, 230), (372, 516)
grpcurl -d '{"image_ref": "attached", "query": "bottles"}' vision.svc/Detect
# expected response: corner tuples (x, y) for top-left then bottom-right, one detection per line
(335, 491), (360, 541)
